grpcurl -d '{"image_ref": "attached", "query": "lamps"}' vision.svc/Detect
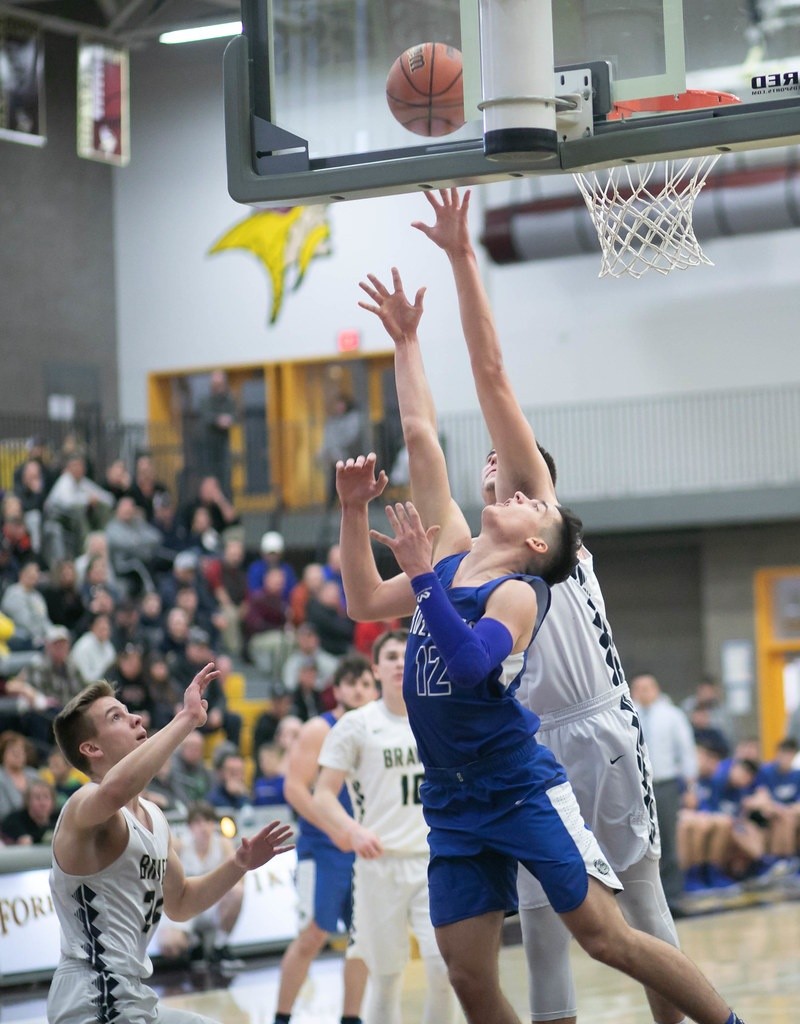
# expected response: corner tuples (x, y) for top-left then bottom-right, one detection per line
(158, 12), (243, 45)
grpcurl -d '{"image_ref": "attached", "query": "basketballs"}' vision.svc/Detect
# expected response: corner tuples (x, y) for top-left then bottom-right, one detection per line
(386, 41), (470, 140)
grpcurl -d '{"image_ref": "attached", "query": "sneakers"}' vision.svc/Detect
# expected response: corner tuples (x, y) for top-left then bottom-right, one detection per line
(681, 853), (799, 896)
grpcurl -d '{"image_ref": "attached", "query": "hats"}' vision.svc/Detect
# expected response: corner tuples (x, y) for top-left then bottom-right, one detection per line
(259, 529), (286, 555)
(174, 549), (199, 568)
(42, 624), (70, 643)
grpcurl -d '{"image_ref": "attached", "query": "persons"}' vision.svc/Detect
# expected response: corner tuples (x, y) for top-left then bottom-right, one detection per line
(631, 670), (800, 918)
(336, 453), (745, 1024)
(312, 628), (457, 1024)
(0, 367), (412, 1002)
(45, 663), (294, 1023)
(358, 186), (688, 1024)
(274, 656), (381, 1024)
